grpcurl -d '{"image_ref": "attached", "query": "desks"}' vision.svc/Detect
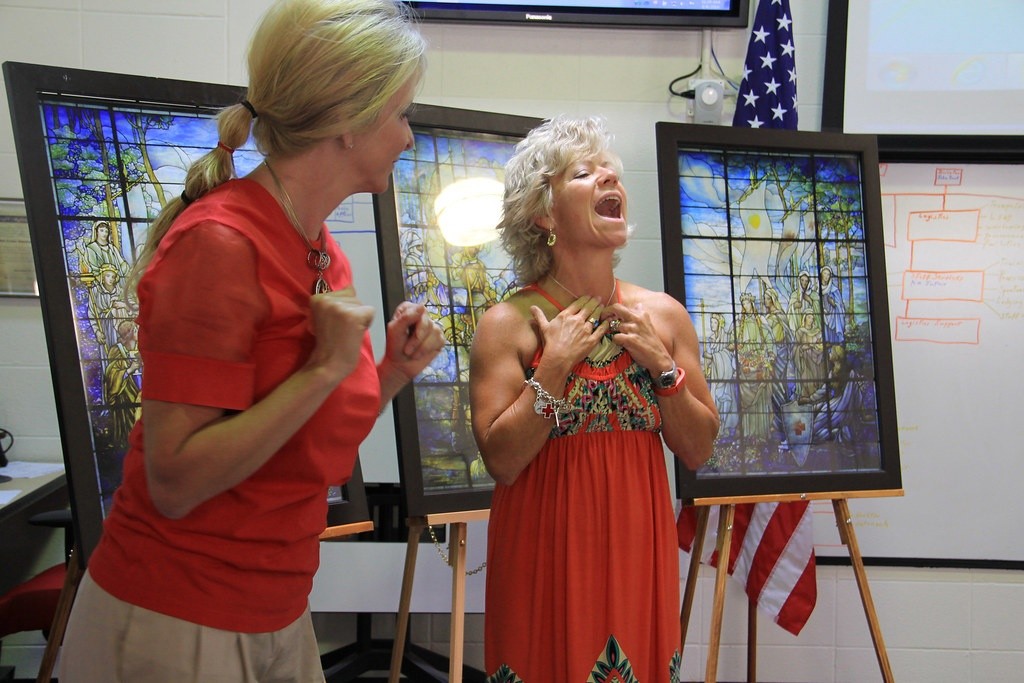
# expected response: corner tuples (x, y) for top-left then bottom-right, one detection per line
(0, 460), (67, 521)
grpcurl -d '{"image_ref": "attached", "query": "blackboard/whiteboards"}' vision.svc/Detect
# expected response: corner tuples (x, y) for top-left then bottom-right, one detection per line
(814, 140), (1024, 568)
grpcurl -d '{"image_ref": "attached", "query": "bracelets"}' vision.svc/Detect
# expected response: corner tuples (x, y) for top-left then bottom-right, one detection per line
(522, 378), (565, 426)
(653, 368), (686, 397)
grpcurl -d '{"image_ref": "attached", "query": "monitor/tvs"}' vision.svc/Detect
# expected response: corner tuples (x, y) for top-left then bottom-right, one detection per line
(398, 0), (749, 28)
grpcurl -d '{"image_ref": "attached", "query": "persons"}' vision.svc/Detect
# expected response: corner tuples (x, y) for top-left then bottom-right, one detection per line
(52, 0), (446, 683)
(471, 116), (719, 683)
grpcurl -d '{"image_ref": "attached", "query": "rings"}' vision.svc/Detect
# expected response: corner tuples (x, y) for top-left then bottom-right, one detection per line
(588, 317), (598, 329)
(610, 320), (620, 331)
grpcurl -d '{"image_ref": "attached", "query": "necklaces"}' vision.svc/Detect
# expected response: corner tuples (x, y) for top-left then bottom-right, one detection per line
(548, 272), (616, 308)
(263, 158), (333, 295)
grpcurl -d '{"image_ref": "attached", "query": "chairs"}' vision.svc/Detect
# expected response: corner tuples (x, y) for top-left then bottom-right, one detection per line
(0, 508), (76, 650)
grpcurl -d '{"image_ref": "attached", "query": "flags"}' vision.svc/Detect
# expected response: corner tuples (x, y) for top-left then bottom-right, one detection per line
(676, 0), (817, 637)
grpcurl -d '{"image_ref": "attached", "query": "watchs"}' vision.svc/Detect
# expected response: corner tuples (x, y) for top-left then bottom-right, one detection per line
(651, 361), (679, 389)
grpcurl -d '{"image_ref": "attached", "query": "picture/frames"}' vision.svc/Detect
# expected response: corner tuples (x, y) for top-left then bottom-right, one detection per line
(369, 101), (551, 521)
(655, 121), (904, 501)
(1, 60), (374, 582)
